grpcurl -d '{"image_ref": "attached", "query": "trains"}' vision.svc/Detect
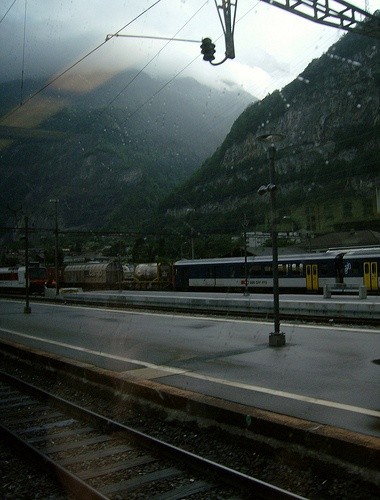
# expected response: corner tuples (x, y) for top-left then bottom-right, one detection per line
(171, 244), (380, 295)
(63, 262), (171, 290)
(0, 267), (48, 295)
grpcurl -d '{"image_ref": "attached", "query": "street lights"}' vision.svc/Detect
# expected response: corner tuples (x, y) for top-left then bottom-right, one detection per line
(19, 214), (31, 314)
(256, 130), (286, 349)
(48, 197), (61, 297)
